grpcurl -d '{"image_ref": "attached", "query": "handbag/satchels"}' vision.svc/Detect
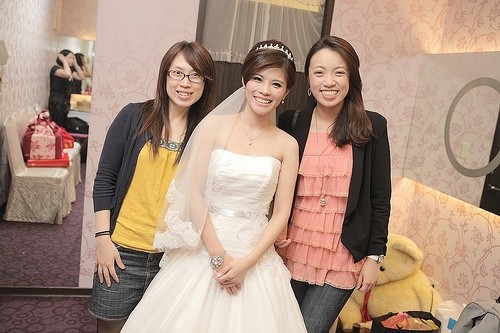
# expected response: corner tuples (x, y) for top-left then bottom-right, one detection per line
(370, 311), (441, 333)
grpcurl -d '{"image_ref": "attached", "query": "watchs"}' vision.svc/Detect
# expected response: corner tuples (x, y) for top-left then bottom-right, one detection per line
(367, 254), (384, 263)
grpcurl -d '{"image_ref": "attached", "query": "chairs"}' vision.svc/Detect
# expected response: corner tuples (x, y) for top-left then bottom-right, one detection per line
(4, 105), (82, 225)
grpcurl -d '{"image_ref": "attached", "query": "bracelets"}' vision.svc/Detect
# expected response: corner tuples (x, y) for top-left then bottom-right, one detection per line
(210, 250), (226, 268)
(94, 231), (112, 238)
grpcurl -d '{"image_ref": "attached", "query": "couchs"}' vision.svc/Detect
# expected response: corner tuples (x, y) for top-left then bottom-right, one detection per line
(388, 179), (500, 333)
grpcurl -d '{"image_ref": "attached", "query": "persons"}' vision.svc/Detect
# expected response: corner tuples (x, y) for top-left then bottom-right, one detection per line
(118, 39), (312, 333)
(91, 40), (219, 333)
(48, 49), (89, 164)
(268, 36), (391, 333)
(67, 52), (91, 95)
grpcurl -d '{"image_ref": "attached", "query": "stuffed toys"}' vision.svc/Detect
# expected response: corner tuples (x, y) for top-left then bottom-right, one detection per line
(338, 234), (446, 330)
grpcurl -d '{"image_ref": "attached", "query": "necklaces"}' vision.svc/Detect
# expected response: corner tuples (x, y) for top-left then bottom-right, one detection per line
(315, 101), (350, 207)
(240, 114), (270, 145)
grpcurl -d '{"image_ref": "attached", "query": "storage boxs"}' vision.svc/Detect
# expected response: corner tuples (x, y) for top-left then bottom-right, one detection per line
(28, 152), (69, 167)
(23, 127), (64, 159)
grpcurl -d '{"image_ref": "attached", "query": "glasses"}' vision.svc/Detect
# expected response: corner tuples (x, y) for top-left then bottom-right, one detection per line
(167, 70), (205, 83)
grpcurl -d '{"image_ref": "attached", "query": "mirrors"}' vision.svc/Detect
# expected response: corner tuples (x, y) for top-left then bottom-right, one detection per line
(402, 53), (500, 215)
(56, 37), (95, 97)
